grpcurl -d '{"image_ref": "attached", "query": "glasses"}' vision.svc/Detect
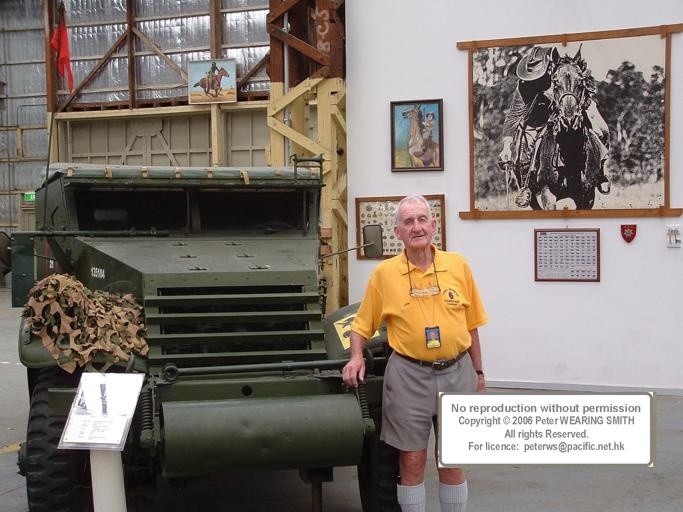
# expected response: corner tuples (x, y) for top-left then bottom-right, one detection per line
(407, 255), (441, 298)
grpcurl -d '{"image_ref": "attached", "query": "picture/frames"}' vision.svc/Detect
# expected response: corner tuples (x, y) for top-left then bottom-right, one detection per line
(457, 23), (683, 221)
(389, 99), (444, 173)
(188, 58), (237, 105)
(535, 228), (601, 283)
(356, 194), (446, 261)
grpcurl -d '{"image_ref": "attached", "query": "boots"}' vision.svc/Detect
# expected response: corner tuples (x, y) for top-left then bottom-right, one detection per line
(597, 161), (611, 194)
(515, 187), (531, 208)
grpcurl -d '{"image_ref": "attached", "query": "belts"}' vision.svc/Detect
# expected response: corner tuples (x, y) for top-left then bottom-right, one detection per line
(394, 350), (468, 371)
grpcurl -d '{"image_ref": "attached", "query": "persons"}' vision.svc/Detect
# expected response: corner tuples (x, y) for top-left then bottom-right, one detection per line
(342, 195), (487, 512)
(499, 46), (612, 207)
(208, 62), (220, 89)
(421, 112), (436, 152)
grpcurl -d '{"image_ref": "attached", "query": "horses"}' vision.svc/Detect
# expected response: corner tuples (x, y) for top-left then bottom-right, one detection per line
(536, 43), (600, 210)
(401, 105), (439, 167)
(192, 68), (229, 99)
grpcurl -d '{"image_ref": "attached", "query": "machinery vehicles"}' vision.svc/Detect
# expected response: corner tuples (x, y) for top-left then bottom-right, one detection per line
(8, 161), (399, 512)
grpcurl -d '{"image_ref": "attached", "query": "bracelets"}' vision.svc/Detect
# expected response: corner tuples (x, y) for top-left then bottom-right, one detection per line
(476, 370), (485, 375)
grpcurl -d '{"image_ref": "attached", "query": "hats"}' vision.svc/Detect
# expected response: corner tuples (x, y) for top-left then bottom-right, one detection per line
(516, 47), (561, 82)
(426, 111), (435, 120)
